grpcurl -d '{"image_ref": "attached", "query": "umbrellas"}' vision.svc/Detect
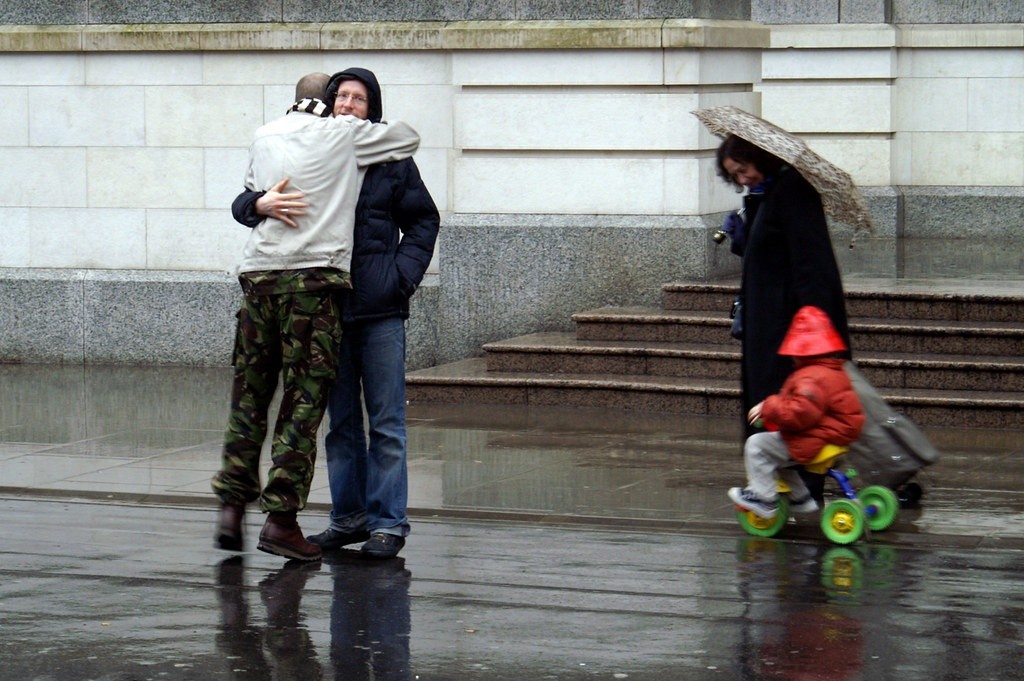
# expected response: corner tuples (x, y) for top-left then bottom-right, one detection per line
(690, 104), (875, 248)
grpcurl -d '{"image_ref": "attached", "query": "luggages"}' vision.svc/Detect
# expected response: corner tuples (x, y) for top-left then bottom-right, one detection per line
(835, 361), (942, 502)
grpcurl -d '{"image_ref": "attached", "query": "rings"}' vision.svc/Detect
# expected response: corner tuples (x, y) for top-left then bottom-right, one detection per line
(282, 208), (288, 213)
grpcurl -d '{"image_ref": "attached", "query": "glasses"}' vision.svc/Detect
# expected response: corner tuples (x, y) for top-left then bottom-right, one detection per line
(333, 91), (370, 104)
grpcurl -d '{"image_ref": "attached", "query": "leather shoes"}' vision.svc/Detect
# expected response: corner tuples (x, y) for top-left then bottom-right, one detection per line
(214, 504), (244, 551)
(258, 512), (322, 560)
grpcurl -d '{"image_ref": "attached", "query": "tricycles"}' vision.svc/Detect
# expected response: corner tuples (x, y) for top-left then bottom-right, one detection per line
(736, 418), (898, 546)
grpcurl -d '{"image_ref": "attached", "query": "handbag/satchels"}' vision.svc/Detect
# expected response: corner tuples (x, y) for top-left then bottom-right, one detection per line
(728, 300), (747, 341)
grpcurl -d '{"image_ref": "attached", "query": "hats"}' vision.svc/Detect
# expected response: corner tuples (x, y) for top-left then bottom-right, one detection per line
(777, 305), (847, 357)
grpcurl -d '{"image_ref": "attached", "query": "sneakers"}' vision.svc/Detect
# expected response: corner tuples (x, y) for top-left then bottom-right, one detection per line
(789, 497), (820, 514)
(362, 529), (406, 558)
(305, 527), (371, 549)
(727, 486), (779, 519)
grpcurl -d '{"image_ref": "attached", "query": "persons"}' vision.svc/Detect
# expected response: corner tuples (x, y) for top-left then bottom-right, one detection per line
(211, 73), (420, 564)
(728, 306), (864, 518)
(715, 133), (850, 507)
(231, 68), (441, 557)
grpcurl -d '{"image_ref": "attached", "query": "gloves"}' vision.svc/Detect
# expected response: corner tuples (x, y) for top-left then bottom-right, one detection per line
(723, 212), (746, 242)
